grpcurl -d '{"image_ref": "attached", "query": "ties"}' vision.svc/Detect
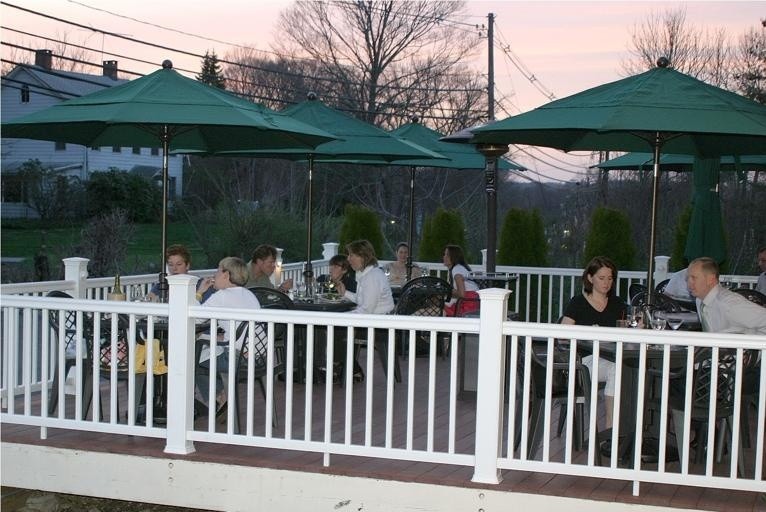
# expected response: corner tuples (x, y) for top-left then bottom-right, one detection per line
(699, 303), (707, 332)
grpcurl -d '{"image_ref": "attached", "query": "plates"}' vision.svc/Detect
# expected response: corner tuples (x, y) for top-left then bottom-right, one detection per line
(317, 293), (346, 303)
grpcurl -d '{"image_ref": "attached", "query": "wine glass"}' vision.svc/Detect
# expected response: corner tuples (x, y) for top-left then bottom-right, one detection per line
(295, 280), (335, 300)
(651, 310), (667, 351)
(668, 319), (684, 350)
(383, 264), (430, 288)
(627, 306), (642, 328)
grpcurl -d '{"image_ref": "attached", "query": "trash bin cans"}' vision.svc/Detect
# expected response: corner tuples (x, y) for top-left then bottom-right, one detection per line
(457, 312), (522, 401)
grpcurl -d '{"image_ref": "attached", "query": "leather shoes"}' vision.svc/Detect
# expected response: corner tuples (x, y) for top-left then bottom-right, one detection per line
(215, 401), (226, 418)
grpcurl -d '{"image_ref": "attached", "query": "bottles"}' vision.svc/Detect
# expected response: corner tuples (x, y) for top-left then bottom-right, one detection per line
(112, 273), (123, 294)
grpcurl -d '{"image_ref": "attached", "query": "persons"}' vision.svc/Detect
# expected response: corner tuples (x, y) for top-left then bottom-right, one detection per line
(410, 244), (479, 318)
(140, 242), (209, 422)
(662, 267), (692, 297)
(379, 241), (420, 288)
(332, 238), (395, 385)
(558, 255), (664, 464)
(674, 256), (765, 458)
(245, 244), (295, 302)
(753, 244), (765, 295)
(193, 253), (267, 424)
(313, 255), (355, 305)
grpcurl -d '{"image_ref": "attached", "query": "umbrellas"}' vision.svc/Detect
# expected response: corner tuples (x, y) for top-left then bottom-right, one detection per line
(169, 89), (451, 296)
(589, 151), (766, 172)
(683, 155), (728, 268)
(300, 113), (529, 269)
(472, 58), (765, 311)
(0, 59), (346, 411)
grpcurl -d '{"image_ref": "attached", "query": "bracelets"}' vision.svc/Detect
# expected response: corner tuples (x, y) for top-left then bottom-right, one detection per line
(197, 293), (204, 303)
